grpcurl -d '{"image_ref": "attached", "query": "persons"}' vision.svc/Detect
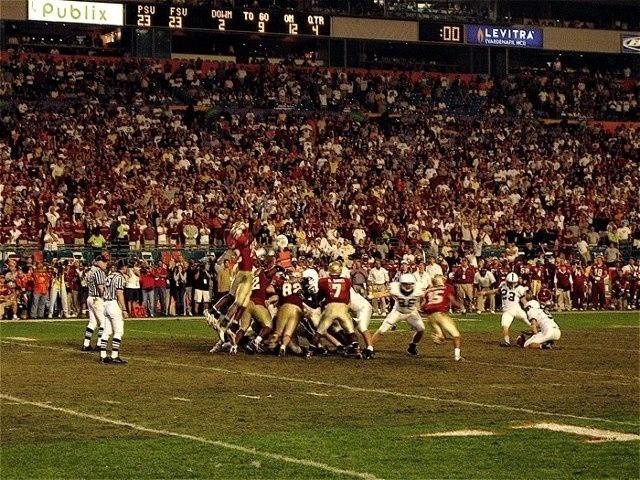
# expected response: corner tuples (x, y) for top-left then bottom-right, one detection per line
(0, 0), (640, 319)
(479, 272), (562, 348)
(367, 273), (467, 361)
(202, 223), (374, 359)
(82, 255), (130, 363)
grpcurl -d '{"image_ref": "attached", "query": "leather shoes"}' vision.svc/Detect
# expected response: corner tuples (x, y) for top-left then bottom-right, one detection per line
(99, 356), (111, 362)
(82, 345), (93, 351)
(111, 357), (128, 364)
(96, 347), (101, 351)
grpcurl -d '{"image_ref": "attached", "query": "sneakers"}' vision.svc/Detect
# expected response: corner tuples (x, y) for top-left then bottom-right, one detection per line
(0, 251), (638, 321)
(406, 348), (420, 358)
(433, 336), (448, 345)
(203, 307), (377, 358)
(498, 338), (555, 350)
(454, 356), (466, 362)
